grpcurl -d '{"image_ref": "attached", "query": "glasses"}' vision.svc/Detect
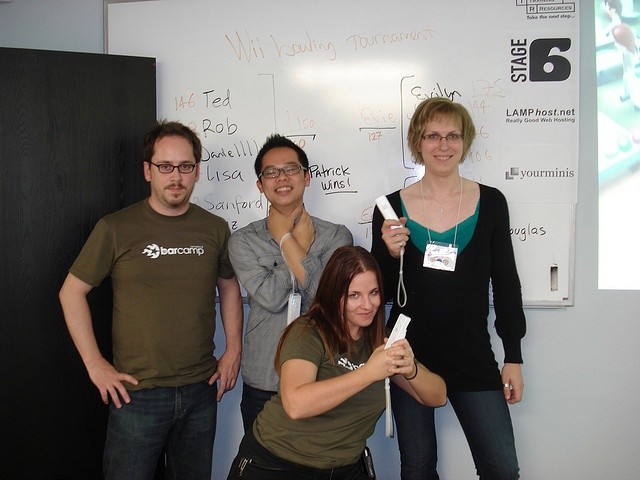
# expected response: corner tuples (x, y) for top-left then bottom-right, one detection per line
(258, 165), (307, 179)
(149, 161), (199, 174)
(421, 134), (463, 141)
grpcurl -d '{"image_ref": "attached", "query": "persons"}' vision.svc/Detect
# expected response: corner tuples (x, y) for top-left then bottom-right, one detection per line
(57, 116), (244, 478)
(228, 134), (354, 436)
(611, 24), (640, 111)
(605, 0), (624, 29)
(371, 98), (526, 480)
(225, 246), (447, 478)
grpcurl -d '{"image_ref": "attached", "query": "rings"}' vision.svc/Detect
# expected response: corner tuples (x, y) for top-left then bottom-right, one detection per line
(504, 383), (509, 387)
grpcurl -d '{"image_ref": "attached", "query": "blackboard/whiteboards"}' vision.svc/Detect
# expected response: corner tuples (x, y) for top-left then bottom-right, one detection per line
(104, 0), (579, 309)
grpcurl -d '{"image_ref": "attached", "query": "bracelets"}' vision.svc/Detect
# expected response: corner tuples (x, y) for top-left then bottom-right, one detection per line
(403, 362), (418, 380)
